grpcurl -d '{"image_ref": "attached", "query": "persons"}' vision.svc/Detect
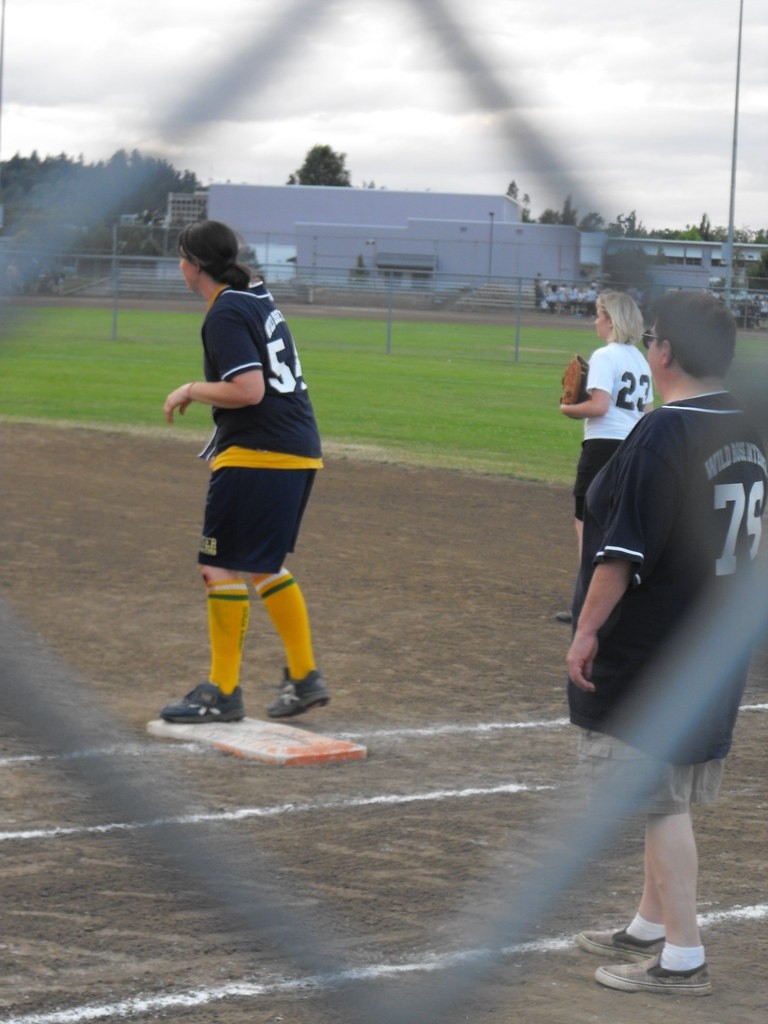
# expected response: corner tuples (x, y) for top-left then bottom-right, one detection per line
(1, 256), (69, 298)
(159, 218), (333, 725)
(534, 272), (768, 330)
(555, 291), (656, 624)
(561, 291), (768, 997)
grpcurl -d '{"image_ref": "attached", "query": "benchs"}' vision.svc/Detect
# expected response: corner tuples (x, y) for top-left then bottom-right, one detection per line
(104, 268), (590, 312)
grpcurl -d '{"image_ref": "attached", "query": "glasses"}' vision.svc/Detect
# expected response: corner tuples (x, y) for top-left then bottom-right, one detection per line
(642, 329), (675, 356)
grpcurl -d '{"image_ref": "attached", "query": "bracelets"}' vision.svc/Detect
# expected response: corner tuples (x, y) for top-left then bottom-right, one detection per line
(187, 381), (197, 400)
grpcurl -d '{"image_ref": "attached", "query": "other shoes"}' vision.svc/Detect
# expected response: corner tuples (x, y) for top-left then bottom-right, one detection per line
(594, 952), (712, 996)
(578, 926), (666, 963)
(556, 610), (573, 623)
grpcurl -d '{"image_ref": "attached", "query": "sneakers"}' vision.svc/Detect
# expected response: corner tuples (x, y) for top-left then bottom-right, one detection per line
(267, 667), (328, 718)
(160, 682), (245, 722)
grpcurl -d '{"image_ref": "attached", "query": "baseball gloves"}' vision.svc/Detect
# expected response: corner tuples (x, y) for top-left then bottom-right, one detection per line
(560, 353), (589, 405)
